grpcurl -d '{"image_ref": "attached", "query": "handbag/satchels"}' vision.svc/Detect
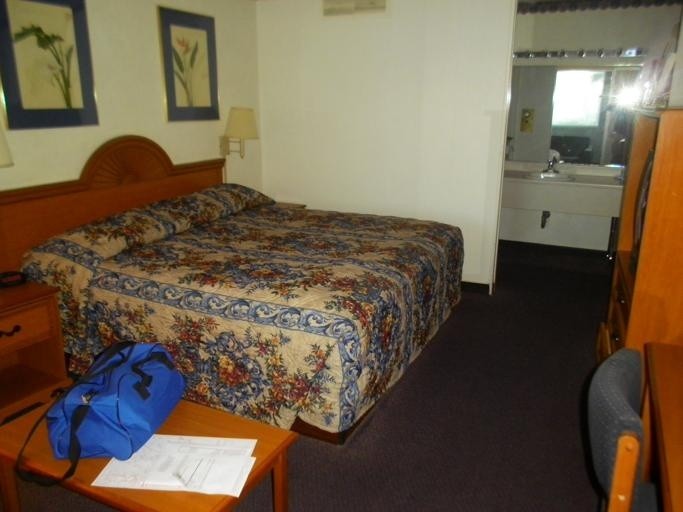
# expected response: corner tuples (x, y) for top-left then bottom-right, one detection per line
(46, 340), (185, 460)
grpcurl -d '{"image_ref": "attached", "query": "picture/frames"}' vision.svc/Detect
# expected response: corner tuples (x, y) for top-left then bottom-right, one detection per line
(0, 0), (99, 131)
(157, 6), (221, 123)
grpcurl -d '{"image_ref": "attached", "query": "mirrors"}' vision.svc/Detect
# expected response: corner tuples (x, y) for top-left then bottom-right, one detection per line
(505, 63), (644, 169)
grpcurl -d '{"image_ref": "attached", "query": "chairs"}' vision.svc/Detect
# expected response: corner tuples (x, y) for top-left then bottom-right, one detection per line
(586, 348), (663, 510)
(550, 135), (594, 164)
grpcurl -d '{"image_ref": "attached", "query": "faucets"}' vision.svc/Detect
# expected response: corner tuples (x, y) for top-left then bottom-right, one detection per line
(542, 160), (560, 173)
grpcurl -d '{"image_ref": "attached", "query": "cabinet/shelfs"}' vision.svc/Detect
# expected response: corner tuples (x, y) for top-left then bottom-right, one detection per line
(595, 105), (683, 366)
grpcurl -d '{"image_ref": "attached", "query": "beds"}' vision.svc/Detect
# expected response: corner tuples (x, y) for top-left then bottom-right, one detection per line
(0, 158), (464, 447)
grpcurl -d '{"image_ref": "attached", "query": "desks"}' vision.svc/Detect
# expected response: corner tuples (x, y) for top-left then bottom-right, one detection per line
(0, 399), (301, 511)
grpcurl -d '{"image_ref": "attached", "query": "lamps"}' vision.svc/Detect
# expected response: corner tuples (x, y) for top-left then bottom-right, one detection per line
(218, 107), (259, 158)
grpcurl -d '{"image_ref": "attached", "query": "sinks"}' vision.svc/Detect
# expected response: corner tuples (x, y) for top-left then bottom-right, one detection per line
(523, 173), (576, 181)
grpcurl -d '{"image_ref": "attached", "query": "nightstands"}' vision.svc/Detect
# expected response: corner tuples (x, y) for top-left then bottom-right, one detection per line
(0, 280), (73, 423)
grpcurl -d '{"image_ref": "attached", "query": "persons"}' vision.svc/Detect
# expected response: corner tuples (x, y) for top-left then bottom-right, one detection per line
(606, 103), (626, 163)
